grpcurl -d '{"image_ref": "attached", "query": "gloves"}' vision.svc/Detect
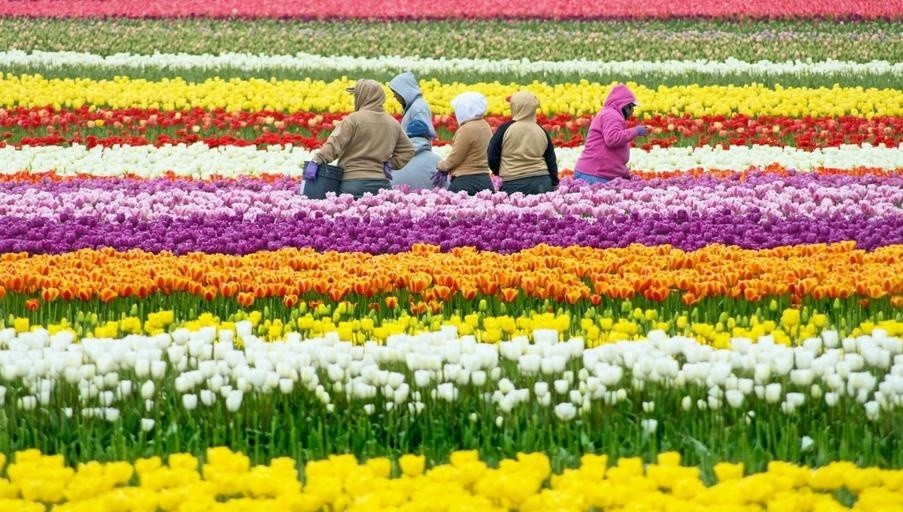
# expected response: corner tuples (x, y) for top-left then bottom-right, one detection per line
(431, 168), (444, 188)
(305, 162), (318, 180)
(637, 125), (649, 137)
(384, 162), (393, 181)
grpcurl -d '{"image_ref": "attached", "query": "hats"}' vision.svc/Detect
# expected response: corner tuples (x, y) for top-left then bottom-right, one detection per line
(633, 99), (640, 107)
(407, 119), (436, 138)
(505, 96), (511, 101)
(346, 87), (356, 93)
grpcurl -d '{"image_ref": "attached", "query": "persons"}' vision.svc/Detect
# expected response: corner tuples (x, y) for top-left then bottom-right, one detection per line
(305, 78), (415, 199)
(573, 84), (648, 184)
(430, 91), (494, 195)
(488, 92), (559, 196)
(388, 73), (437, 158)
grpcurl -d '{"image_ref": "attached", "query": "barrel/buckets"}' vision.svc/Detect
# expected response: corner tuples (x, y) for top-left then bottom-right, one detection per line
(299, 160), (344, 197)
